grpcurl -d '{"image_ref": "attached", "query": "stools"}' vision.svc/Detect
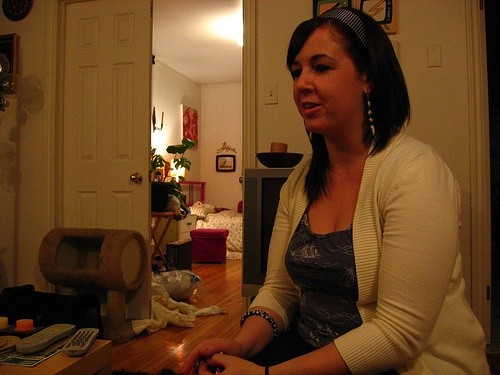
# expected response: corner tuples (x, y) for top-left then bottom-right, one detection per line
(189, 229), (228, 264)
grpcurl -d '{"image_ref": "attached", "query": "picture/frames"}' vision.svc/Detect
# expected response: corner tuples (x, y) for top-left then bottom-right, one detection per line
(180, 104), (199, 150)
(216, 154), (235, 172)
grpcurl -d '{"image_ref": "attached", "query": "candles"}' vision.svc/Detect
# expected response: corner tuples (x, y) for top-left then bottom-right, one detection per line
(0, 317), (8, 329)
(16, 318), (34, 331)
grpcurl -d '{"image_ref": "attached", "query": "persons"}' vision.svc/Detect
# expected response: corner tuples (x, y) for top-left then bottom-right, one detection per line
(180, 5), (492, 375)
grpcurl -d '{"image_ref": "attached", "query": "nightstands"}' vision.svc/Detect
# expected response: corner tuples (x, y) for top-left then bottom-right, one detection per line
(155, 215), (195, 252)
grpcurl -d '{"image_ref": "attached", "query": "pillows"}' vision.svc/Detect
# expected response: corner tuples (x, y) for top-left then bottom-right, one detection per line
(189, 205), (212, 218)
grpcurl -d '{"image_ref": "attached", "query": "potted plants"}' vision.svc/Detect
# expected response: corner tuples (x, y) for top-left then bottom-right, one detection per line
(150, 137), (196, 219)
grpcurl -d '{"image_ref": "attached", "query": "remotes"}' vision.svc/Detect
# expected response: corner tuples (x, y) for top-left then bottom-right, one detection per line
(16, 324), (75, 354)
(62, 328), (99, 357)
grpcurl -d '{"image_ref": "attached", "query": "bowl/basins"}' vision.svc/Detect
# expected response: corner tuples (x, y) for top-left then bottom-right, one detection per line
(256, 152), (304, 168)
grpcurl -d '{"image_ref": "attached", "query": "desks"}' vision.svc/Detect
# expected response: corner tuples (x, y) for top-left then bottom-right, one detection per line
(151, 210), (175, 266)
(0, 339), (113, 375)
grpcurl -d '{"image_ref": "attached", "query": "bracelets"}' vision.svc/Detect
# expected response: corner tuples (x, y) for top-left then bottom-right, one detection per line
(265, 364), (269, 375)
(240, 309), (279, 342)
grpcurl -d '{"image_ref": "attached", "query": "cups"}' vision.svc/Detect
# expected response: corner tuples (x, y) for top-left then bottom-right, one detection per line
(270, 142), (288, 152)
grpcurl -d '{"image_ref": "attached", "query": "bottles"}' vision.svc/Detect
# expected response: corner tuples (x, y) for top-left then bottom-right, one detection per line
(238, 201), (242, 212)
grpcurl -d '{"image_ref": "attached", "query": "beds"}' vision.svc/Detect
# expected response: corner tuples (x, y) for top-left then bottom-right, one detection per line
(180, 180), (242, 259)
